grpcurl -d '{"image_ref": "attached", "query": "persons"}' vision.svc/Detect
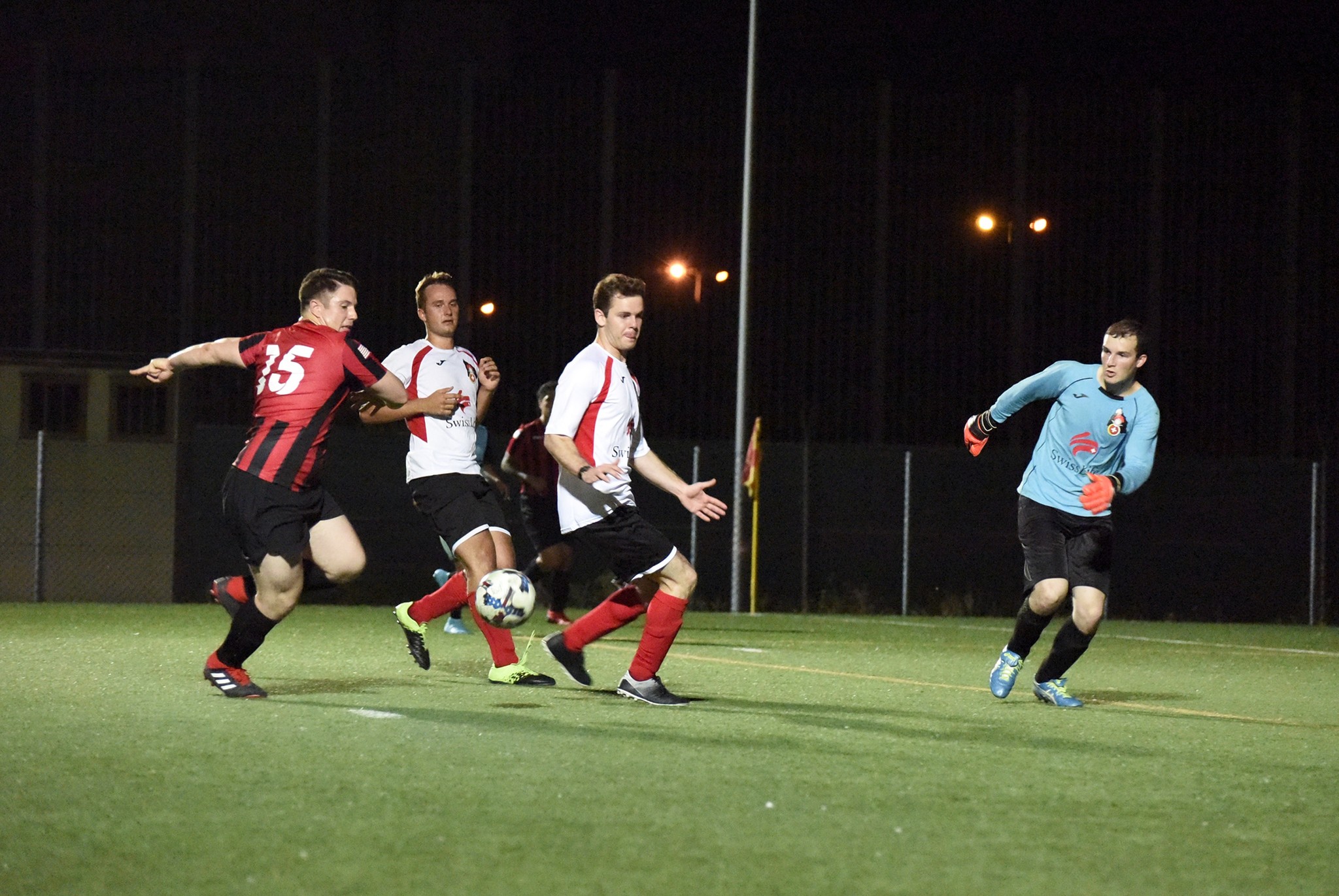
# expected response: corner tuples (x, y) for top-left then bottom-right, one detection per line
(542, 272), (727, 707)
(501, 379), (578, 624)
(432, 425), (510, 634)
(129, 269), (407, 698)
(966, 318), (1161, 713)
(348, 271), (556, 686)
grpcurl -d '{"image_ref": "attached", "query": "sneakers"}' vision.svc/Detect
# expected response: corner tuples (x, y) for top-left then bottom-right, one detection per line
(433, 569), (451, 587)
(540, 629), (592, 686)
(393, 600), (430, 671)
(989, 643), (1024, 697)
(1032, 671), (1084, 708)
(616, 670), (691, 706)
(443, 615), (472, 635)
(210, 576), (243, 619)
(204, 653), (268, 700)
(487, 630), (556, 686)
(546, 610), (575, 627)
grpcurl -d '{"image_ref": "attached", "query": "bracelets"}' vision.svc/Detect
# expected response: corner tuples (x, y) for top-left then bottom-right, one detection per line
(578, 466), (591, 481)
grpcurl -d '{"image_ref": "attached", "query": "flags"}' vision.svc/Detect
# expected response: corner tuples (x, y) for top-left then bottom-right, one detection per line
(742, 424), (761, 497)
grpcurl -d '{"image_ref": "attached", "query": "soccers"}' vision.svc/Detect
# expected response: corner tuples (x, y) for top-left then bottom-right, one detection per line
(474, 568), (536, 630)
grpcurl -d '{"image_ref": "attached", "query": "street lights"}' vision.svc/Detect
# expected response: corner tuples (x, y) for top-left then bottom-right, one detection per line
(667, 262), (730, 305)
(975, 214), (1048, 246)
